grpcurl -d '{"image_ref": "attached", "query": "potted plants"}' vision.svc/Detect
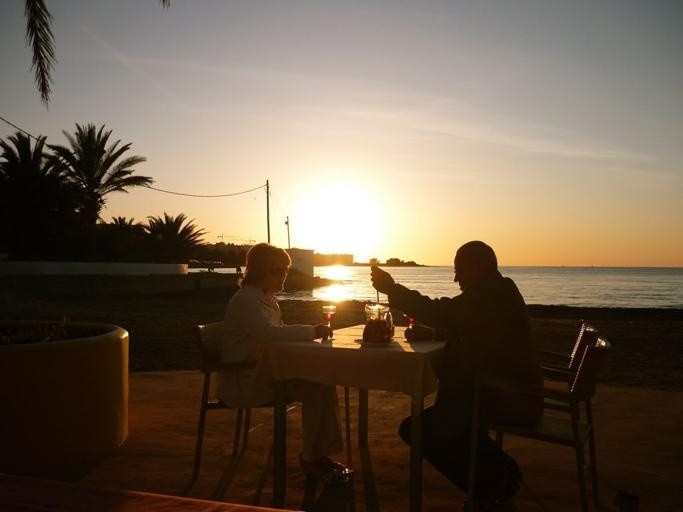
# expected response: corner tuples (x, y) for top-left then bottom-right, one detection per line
(0, 318), (127, 491)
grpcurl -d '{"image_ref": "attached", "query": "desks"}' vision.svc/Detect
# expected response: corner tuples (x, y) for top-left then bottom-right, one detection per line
(265, 323), (445, 506)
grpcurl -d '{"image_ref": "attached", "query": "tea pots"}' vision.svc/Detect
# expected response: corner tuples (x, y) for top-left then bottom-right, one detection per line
(362, 303), (394, 344)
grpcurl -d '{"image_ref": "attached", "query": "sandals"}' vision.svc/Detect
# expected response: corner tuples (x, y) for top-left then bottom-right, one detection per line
(299, 452), (354, 488)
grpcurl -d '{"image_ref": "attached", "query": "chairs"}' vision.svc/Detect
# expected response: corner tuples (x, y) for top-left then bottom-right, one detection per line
(539, 323), (600, 391)
(193, 323), (294, 476)
(477, 337), (607, 505)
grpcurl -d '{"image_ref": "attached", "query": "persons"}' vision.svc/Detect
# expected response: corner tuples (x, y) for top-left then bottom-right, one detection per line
(215, 242), (355, 487)
(371, 240), (545, 512)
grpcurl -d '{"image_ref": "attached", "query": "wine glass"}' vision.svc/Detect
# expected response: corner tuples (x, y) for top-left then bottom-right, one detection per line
(404, 311), (419, 342)
(321, 305), (337, 340)
